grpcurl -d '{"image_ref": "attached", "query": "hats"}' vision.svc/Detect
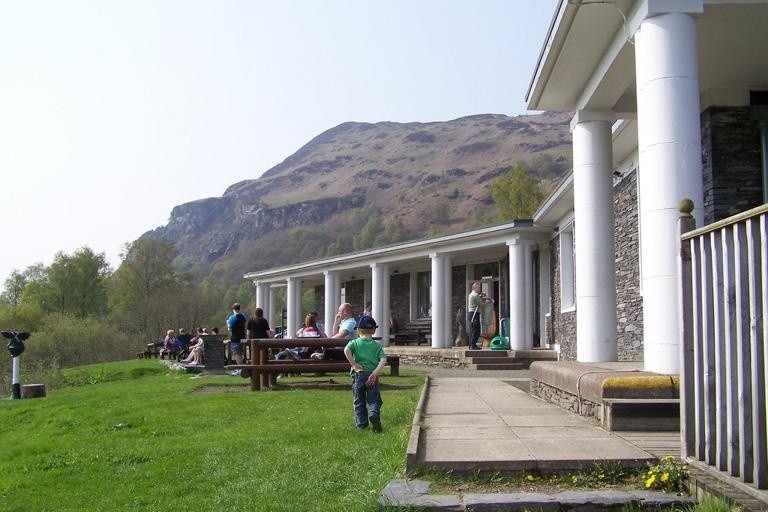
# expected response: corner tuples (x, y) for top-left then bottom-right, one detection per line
(353, 316), (378, 330)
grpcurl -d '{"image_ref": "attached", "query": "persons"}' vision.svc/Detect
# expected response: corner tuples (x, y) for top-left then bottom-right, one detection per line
(246, 308), (269, 337)
(275, 301), (371, 378)
(163, 327), (219, 365)
(344, 315), (387, 433)
(226, 302), (248, 375)
(468, 281), (490, 350)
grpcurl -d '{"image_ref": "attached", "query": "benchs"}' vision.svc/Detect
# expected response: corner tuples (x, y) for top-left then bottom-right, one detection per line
(330, 352), (402, 376)
(247, 358), (350, 387)
(142, 338), (227, 364)
(393, 317), (431, 345)
(224, 363), (352, 390)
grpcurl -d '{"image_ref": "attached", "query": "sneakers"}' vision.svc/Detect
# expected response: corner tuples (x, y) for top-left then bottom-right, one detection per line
(469, 345), (480, 350)
(369, 412), (382, 433)
(231, 369), (241, 375)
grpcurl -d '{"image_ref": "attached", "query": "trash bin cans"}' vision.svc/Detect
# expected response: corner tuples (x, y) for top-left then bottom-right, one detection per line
(500, 314), (510, 350)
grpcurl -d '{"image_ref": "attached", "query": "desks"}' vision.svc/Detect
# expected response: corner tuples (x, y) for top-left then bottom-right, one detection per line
(239, 337), (354, 390)
